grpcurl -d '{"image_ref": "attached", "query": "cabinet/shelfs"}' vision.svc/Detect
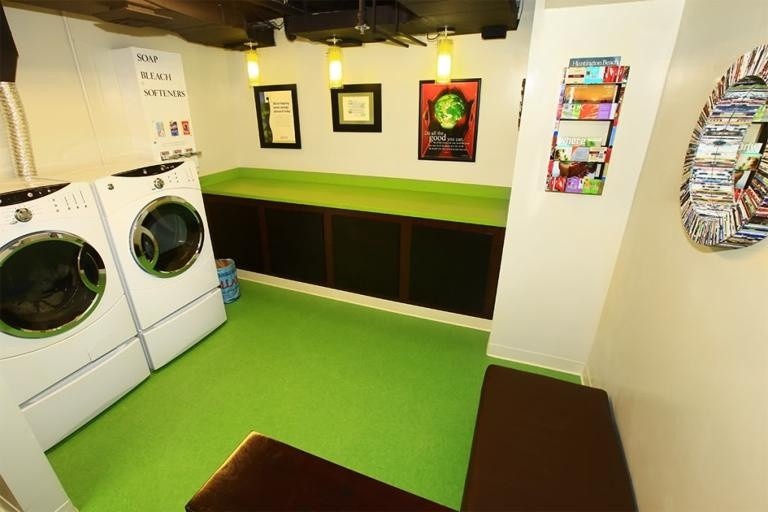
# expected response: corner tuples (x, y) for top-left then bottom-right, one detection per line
(201, 176), (511, 332)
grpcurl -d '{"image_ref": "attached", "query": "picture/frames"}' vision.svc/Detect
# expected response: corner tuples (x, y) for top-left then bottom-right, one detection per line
(253, 83), (302, 150)
(330, 83), (382, 133)
(417, 77), (482, 163)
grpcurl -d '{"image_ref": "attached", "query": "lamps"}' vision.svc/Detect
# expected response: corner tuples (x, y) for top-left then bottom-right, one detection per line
(435, 25), (456, 86)
(242, 42), (262, 86)
(327, 37), (345, 90)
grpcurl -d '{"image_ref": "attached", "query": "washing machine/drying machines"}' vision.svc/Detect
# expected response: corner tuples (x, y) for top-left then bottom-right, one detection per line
(0, 179), (152, 455)
(93, 157), (227, 373)
